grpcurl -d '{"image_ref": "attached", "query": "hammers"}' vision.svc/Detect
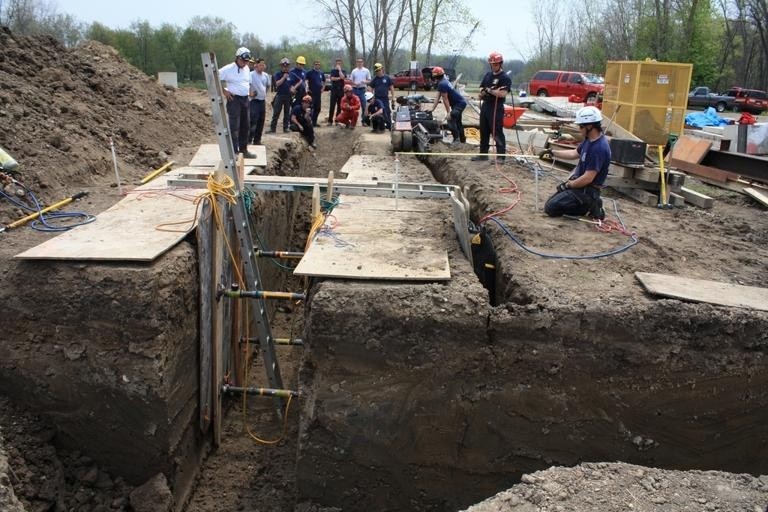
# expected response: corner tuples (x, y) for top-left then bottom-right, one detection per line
(656, 144), (673, 210)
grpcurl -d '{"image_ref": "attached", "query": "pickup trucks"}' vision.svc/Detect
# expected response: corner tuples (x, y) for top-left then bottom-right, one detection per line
(671, 85), (736, 112)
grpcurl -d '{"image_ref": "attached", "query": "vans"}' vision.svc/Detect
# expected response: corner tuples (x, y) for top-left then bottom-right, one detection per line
(391, 70), (431, 90)
(528, 70), (605, 108)
(727, 86), (768, 113)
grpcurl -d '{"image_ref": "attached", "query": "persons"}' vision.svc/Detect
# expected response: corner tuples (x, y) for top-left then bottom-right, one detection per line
(424, 67), (467, 149)
(470, 51), (512, 165)
(538, 105), (612, 220)
(218, 47), (397, 158)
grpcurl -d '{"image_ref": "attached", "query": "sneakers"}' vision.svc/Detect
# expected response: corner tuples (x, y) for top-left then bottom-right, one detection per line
(314, 123), (321, 126)
(592, 199), (604, 221)
(311, 142), (317, 148)
(371, 130), (385, 133)
(239, 152), (256, 158)
(265, 131), (276, 134)
(326, 122), (354, 129)
(283, 129), (291, 133)
(450, 140), (466, 145)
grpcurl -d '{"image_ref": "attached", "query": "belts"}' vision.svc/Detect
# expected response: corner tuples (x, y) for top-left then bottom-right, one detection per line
(233, 95), (249, 98)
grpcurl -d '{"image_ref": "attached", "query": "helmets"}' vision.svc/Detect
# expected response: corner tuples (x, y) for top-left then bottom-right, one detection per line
(364, 92), (373, 100)
(374, 63), (383, 72)
(234, 47), (264, 64)
(343, 84), (352, 92)
(296, 57), (306, 64)
(432, 67), (444, 77)
(280, 58), (290, 64)
(573, 106), (602, 123)
(489, 52), (502, 64)
(303, 96), (311, 102)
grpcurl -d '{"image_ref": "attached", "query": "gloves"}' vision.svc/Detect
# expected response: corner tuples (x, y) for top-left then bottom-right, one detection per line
(499, 86), (508, 90)
(446, 112), (451, 120)
(483, 88), (491, 93)
(556, 181), (568, 192)
(539, 148), (554, 159)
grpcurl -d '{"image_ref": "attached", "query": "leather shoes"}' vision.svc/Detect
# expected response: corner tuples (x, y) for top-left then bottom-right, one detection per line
(471, 156), (488, 161)
(491, 160), (505, 165)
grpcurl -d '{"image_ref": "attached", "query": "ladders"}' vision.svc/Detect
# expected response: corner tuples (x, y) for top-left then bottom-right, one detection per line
(168, 174), (462, 200)
(202, 50), (288, 426)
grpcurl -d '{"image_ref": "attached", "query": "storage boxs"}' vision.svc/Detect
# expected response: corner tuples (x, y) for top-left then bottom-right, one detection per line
(601, 59), (693, 145)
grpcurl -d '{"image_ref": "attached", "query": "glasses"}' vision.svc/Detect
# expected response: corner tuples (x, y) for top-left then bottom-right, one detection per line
(579, 124), (592, 128)
